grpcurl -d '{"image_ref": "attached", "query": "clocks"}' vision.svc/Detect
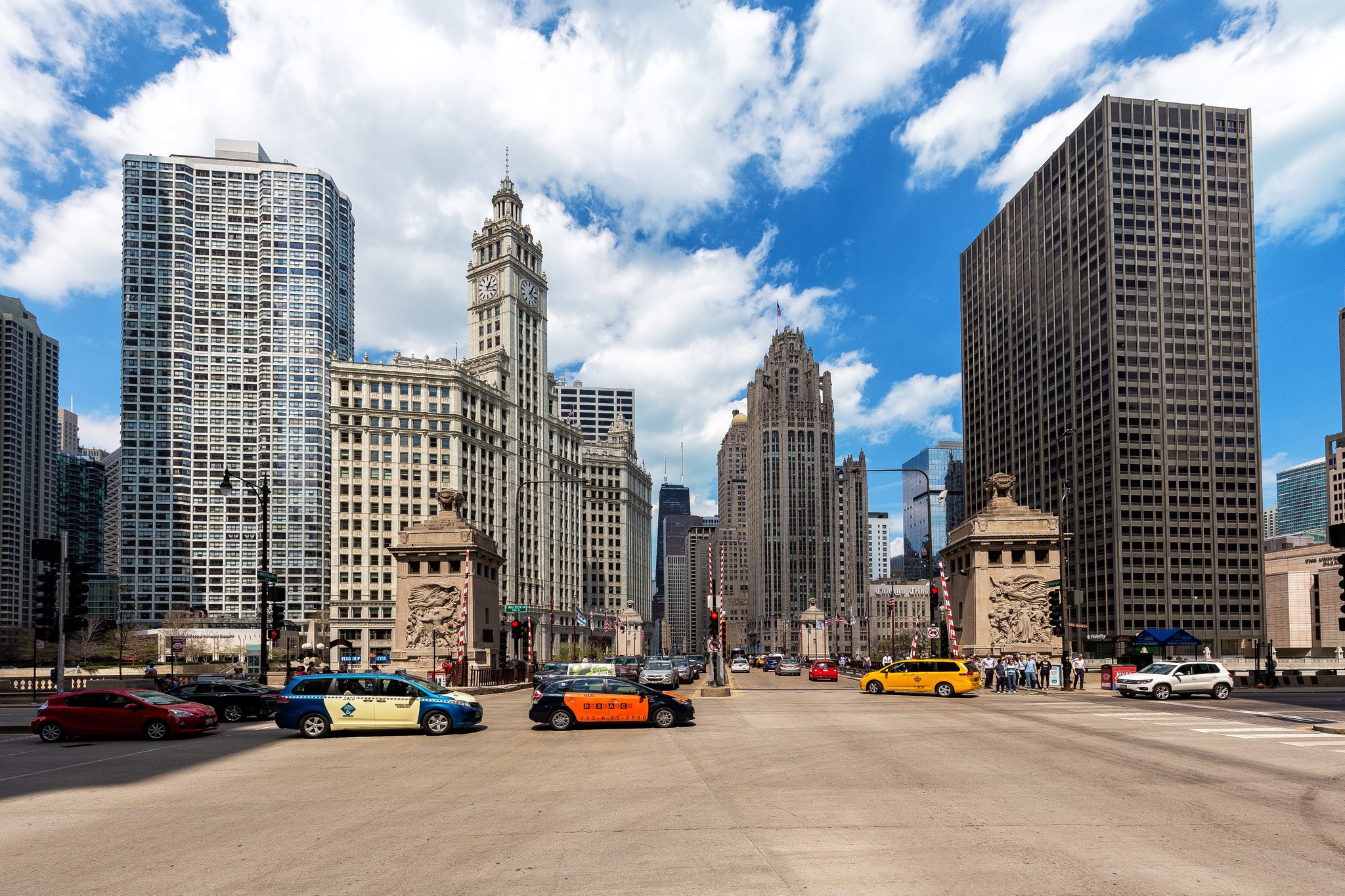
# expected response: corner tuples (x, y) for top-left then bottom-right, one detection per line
(521, 281), (538, 306)
(478, 275), (497, 301)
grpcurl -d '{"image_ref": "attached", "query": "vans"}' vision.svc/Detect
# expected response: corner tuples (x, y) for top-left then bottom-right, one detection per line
(275, 672), (483, 737)
(859, 658), (982, 698)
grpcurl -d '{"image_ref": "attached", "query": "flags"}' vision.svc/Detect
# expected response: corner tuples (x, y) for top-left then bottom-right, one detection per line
(550, 600), (645, 641)
(808, 587), (895, 634)
(777, 302), (781, 317)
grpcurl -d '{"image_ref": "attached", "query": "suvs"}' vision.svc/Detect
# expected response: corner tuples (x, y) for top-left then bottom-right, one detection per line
(732, 655), (750, 670)
(1116, 660), (1234, 700)
(532, 655), (707, 690)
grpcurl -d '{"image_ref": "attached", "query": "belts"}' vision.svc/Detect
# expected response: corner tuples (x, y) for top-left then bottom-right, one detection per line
(1075, 668), (1083, 670)
(447, 673), (451, 674)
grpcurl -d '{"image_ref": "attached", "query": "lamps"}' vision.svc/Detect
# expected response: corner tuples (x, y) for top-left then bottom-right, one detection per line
(301, 643), (325, 658)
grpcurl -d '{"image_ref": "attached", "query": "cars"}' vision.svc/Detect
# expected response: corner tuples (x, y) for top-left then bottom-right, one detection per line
(528, 662), (696, 731)
(749, 654), (788, 672)
(162, 680), (282, 723)
(30, 689), (220, 743)
(808, 661), (838, 682)
(731, 658), (749, 673)
(775, 659), (800, 677)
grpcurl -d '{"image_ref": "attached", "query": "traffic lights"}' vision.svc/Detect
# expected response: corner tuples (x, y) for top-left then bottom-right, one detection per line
(940, 621), (950, 657)
(1053, 627), (1064, 636)
(721, 620), (727, 659)
(727, 649), (728, 654)
(1048, 589), (1060, 626)
(499, 630), (508, 669)
(511, 621), (523, 639)
(758, 638), (760, 643)
(930, 586), (939, 607)
(33, 573), (57, 642)
(710, 612), (719, 635)
(71, 557), (91, 632)
(268, 584), (285, 640)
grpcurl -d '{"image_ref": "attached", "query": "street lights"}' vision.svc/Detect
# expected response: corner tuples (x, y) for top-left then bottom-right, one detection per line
(845, 467), (948, 667)
(735, 635), (744, 652)
(295, 646), (298, 659)
(771, 614), (787, 655)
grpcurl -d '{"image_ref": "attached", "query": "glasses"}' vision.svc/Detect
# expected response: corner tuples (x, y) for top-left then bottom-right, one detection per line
(1009, 661), (1013, 662)
(1000, 662), (1003, 663)
(1045, 658), (1048, 659)
(1020, 658), (1023, 659)
(444, 662), (447, 663)
(987, 655), (991, 656)
(297, 667), (299, 669)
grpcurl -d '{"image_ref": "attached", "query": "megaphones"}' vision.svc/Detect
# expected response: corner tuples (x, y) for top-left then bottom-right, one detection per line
(944, 576), (952, 583)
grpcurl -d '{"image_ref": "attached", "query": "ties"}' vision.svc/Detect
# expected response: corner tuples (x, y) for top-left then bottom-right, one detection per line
(887, 657), (888, 663)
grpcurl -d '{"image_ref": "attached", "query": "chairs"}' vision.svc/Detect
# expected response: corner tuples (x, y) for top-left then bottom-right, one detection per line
(108, 694), (118, 702)
(121, 696), (131, 705)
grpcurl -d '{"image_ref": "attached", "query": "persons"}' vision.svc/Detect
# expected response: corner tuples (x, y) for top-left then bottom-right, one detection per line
(787, 654), (1086, 693)
(988, 574), (1052, 645)
(77, 665), (80, 673)
(1167, 656), (1188, 660)
(405, 587), (461, 648)
(141, 660), (245, 693)
(289, 660), (453, 696)
(1264, 655), (1277, 688)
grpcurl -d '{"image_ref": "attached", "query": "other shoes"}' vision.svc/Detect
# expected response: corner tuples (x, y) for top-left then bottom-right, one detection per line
(1038, 687), (1042, 689)
(1033, 688), (1036, 690)
(1042, 688), (1044, 690)
(1073, 687), (1076, 689)
(997, 685), (1027, 693)
(985, 687), (993, 689)
(1046, 688), (1049, 691)
(1079, 688), (1086, 690)
(1027, 688), (1030, 691)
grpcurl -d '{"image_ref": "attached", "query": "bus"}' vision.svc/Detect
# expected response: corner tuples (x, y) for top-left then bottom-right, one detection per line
(731, 648), (745, 662)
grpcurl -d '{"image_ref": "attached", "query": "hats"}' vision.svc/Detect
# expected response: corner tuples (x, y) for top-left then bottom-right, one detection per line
(1001, 656), (1006, 659)
(444, 660), (449, 663)
(286, 662), (292, 665)
